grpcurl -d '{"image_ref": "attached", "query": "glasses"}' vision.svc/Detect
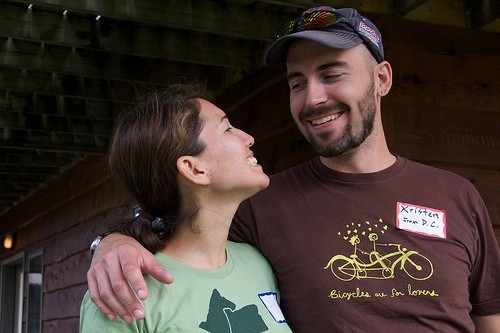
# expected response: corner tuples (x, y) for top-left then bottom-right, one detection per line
(274, 10), (384, 65)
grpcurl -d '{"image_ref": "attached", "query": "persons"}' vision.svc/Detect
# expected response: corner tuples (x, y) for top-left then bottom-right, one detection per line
(78, 81), (292, 333)
(85, 5), (500, 332)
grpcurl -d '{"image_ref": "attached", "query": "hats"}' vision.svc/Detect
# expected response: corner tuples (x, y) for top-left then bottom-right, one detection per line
(263, 5), (385, 68)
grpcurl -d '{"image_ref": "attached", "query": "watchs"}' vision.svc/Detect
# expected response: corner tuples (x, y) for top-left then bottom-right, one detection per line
(90, 228), (130, 258)
